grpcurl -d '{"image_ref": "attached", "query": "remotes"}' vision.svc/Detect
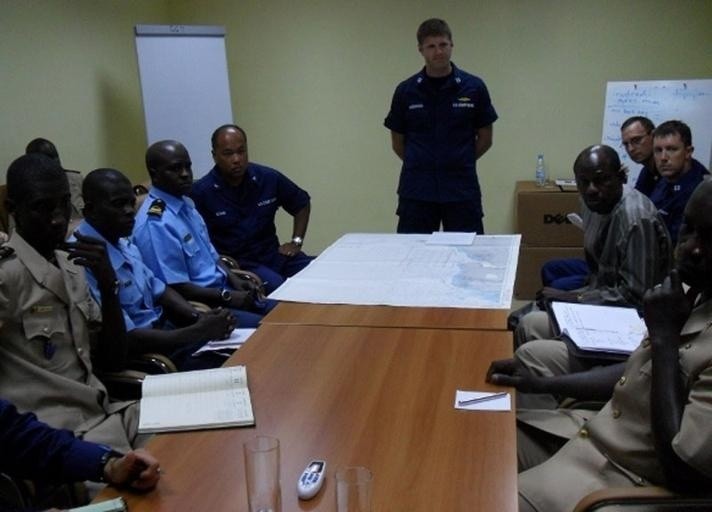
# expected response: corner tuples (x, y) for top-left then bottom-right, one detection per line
(297, 460), (326, 499)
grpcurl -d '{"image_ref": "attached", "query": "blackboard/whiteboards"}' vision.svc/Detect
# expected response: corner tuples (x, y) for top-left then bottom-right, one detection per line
(134, 24), (233, 184)
(601, 79), (711, 191)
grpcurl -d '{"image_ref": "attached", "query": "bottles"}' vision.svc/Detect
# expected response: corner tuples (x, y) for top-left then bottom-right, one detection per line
(534, 153), (545, 187)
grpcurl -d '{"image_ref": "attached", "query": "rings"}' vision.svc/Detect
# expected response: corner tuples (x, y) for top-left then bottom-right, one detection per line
(653, 283), (661, 289)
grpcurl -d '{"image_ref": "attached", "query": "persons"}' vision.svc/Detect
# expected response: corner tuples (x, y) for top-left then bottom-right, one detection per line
(63, 166), (236, 391)
(132, 139), (282, 335)
(619, 116), (661, 203)
(25, 137), (87, 246)
(484, 176), (712, 512)
(382, 17), (500, 236)
(0, 398), (161, 512)
(507, 138), (677, 336)
(650, 120), (712, 252)
(186, 123), (316, 304)
(0, 151), (153, 507)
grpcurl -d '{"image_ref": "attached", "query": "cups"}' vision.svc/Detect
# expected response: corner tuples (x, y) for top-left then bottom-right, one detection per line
(334, 466), (374, 512)
(242, 435), (282, 512)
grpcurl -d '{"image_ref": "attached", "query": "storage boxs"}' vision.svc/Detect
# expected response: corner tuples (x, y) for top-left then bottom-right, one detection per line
(516, 180), (589, 246)
(514, 247), (585, 300)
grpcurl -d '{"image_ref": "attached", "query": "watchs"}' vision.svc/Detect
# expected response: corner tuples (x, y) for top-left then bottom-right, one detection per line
(96, 448), (126, 489)
(191, 308), (202, 322)
(217, 287), (234, 309)
(96, 279), (121, 297)
(291, 235), (305, 245)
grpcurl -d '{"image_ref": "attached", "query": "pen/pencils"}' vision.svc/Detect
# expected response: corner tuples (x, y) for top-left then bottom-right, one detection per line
(459, 392), (508, 406)
(96, 443), (166, 475)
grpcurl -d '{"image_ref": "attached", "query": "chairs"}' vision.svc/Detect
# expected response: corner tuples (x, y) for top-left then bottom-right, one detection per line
(564, 398), (712, 512)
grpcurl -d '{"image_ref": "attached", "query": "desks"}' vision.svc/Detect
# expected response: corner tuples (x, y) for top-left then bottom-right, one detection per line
(86, 324), (517, 512)
(259, 233), (522, 329)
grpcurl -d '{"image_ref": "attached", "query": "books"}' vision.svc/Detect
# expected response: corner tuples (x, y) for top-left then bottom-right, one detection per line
(548, 298), (650, 361)
(136, 364), (256, 435)
(427, 231), (480, 248)
(193, 326), (255, 355)
